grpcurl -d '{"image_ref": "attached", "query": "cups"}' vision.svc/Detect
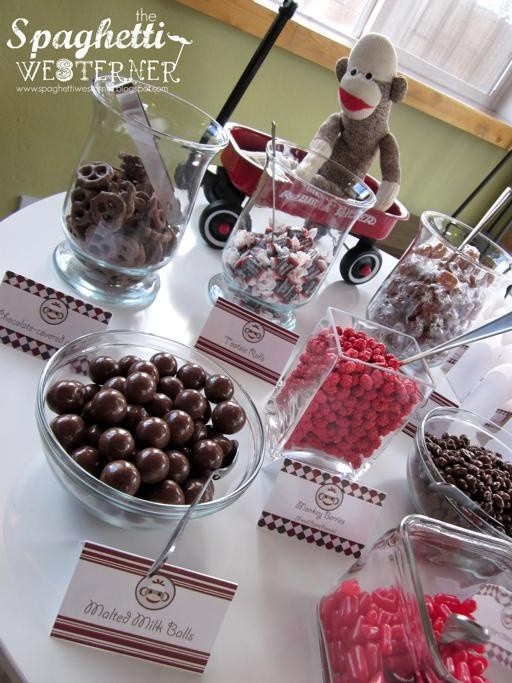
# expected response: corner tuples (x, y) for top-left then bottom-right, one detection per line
(42, 74), (511, 682)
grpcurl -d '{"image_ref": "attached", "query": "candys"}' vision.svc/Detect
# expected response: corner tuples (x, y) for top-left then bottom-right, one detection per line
(46, 153), (512, 683)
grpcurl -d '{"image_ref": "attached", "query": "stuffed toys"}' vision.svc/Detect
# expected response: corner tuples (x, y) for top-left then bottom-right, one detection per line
(294, 30), (408, 214)
